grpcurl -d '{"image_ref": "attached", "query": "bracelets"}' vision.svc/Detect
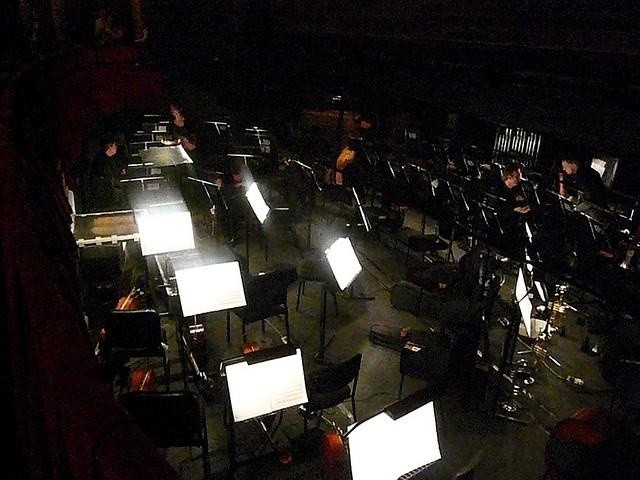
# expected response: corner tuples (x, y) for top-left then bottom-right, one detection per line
(559, 180), (564, 184)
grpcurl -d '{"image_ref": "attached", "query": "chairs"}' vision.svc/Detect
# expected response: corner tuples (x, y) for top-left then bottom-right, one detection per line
(75, 112), (639, 480)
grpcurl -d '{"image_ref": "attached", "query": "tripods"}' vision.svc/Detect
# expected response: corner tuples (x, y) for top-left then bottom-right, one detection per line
(295, 180), (315, 259)
(312, 271), (338, 369)
(444, 214), (463, 266)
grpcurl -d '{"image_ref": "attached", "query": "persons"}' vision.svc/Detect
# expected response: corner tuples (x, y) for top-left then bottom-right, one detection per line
(557, 152), (610, 206)
(90, 137), (127, 210)
(496, 165), (538, 260)
(163, 100), (244, 246)
